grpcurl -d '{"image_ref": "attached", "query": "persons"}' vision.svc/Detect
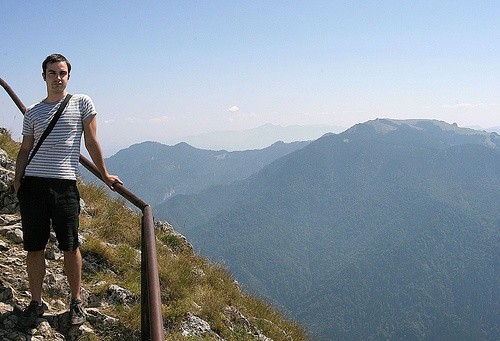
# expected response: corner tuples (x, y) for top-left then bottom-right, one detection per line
(14, 54), (124, 330)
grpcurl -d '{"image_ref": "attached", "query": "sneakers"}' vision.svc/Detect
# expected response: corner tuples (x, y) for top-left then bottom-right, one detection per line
(18, 301), (45, 328)
(71, 299), (86, 325)
